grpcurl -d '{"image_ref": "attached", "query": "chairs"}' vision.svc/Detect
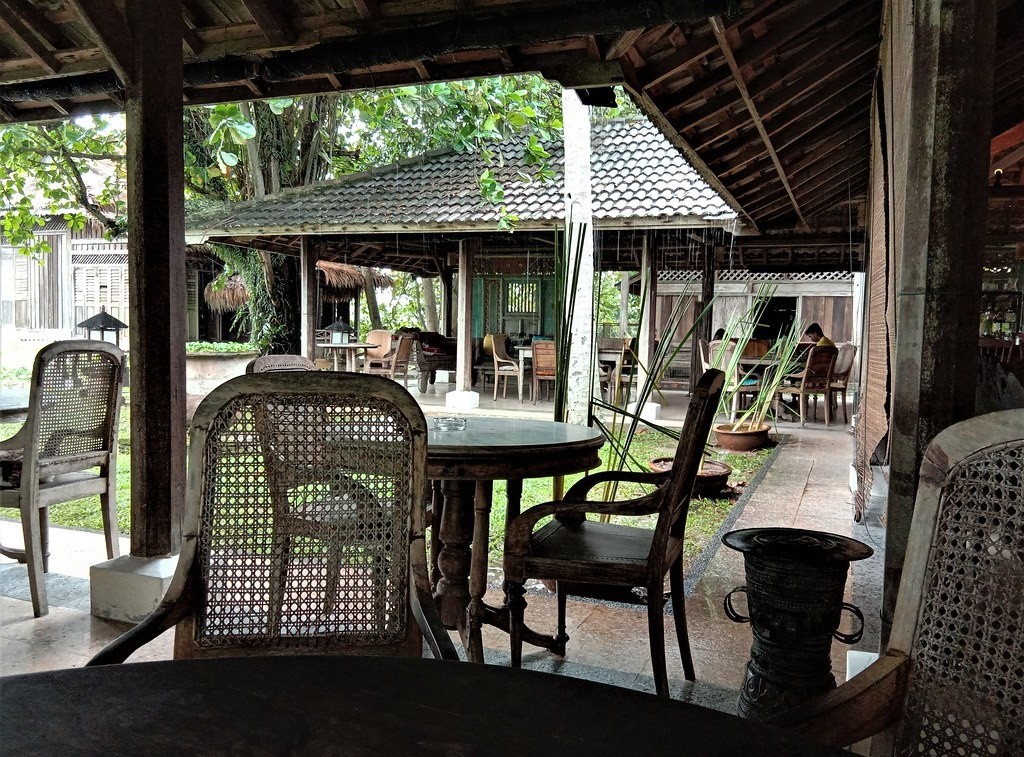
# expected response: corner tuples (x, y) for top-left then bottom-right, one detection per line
(0, 327), (1024, 757)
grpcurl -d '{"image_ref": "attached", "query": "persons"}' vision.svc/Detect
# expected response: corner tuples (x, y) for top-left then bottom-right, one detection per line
(804, 322), (834, 346)
(712, 328), (729, 342)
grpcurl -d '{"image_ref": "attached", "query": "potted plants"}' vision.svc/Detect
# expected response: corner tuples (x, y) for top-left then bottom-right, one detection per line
(529, 202), (811, 592)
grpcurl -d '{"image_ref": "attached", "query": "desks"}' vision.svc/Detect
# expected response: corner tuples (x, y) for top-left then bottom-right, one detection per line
(317, 342), (380, 373)
(513, 345), (533, 403)
(598, 349), (632, 405)
(321, 414), (605, 664)
(1, 653), (866, 757)
(0, 394), (29, 416)
(736, 357), (804, 422)
